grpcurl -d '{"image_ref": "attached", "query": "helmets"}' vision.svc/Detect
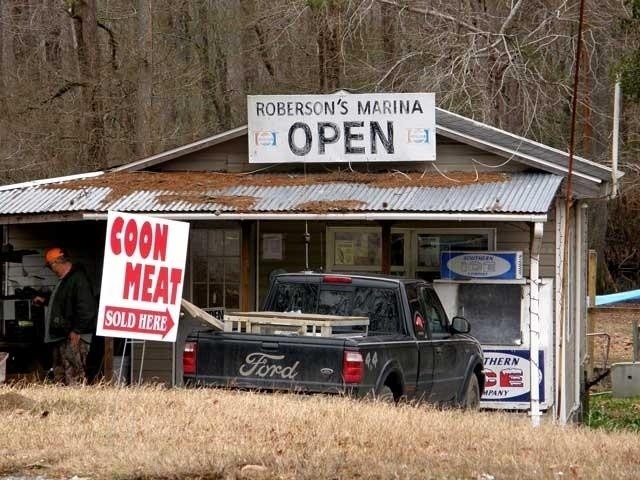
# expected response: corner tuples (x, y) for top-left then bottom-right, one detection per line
(45, 248), (64, 262)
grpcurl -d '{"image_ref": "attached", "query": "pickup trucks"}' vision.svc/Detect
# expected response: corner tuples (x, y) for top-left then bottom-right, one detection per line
(183, 268), (488, 415)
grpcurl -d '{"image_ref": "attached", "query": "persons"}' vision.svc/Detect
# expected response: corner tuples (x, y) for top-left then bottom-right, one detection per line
(33, 247), (97, 386)
